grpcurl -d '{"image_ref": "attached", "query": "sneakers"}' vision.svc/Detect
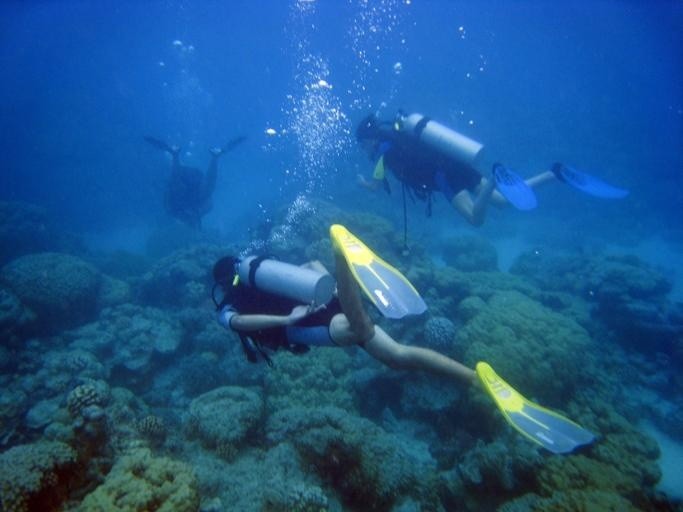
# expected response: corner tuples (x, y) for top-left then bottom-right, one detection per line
(491, 161), (519, 187)
(473, 361), (524, 413)
(549, 160), (589, 187)
(328, 223), (373, 267)
(162, 144), (182, 156)
(208, 145), (228, 158)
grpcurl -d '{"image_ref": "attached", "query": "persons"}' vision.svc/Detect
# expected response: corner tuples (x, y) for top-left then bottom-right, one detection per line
(353, 111), (631, 228)
(138, 132), (248, 235)
(211, 223), (594, 455)
